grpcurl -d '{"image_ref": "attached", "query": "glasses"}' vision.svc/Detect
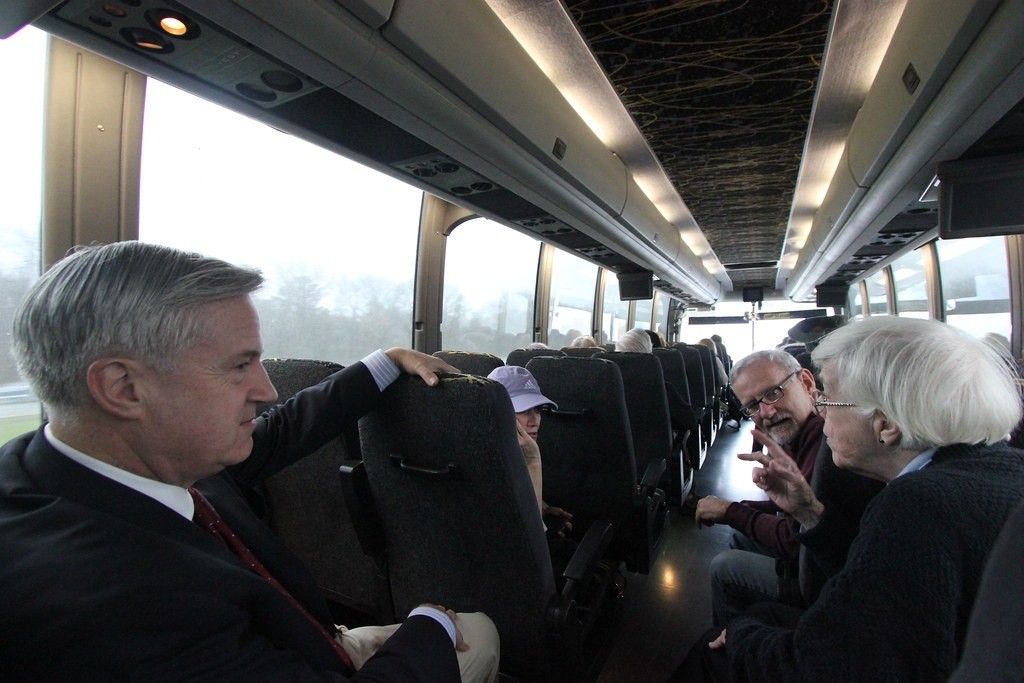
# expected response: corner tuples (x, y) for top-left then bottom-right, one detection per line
(810, 390), (861, 418)
(737, 371), (803, 419)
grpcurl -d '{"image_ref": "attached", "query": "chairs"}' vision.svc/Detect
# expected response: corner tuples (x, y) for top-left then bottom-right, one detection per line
(433, 351), (505, 378)
(592, 352), (694, 507)
(597, 345), (614, 354)
(783, 345), (813, 373)
(670, 348), (717, 448)
(357, 373), (616, 683)
(505, 348), (569, 368)
(799, 431), (887, 610)
(524, 356), (671, 574)
(652, 348), (707, 470)
(946, 499), (1024, 683)
(709, 349), (727, 417)
(560, 347), (606, 357)
(254, 358), (395, 630)
(687, 344), (724, 431)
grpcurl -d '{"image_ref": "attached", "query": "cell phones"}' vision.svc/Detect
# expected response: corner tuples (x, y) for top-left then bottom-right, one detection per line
(542, 512), (569, 539)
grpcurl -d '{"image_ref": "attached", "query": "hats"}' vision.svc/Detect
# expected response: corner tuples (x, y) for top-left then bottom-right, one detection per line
(486, 365), (559, 412)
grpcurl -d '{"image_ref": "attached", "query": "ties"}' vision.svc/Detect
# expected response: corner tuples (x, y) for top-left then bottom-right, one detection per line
(188, 486), (360, 669)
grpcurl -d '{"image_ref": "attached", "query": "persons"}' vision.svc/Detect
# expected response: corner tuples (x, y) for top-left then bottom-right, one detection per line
(0, 242), (502, 683)
(530, 328), (728, 389)
(727, 336), (796, 448)
(675, 315), (1023, 681)
(695, 351), (884, 628)
(485, 363), (573, 538)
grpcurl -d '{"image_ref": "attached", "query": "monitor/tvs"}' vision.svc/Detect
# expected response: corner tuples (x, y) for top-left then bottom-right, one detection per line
(617, 271), (653, 301)
(938, 152), (1024, 239)
(743, 287), (763, 302)
(816, 285), (849, 307)
